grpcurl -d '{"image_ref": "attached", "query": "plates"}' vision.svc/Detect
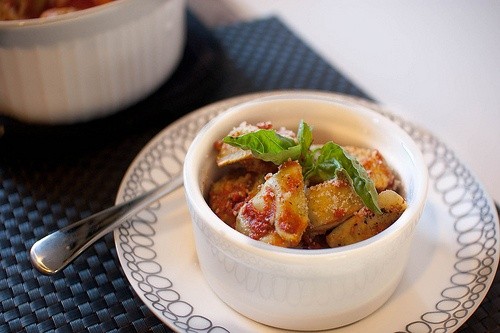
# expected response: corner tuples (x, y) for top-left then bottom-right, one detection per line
(112, 89), (499, 333)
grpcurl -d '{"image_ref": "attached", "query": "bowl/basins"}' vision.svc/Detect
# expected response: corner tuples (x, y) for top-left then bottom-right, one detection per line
(0, 0), (187, 121)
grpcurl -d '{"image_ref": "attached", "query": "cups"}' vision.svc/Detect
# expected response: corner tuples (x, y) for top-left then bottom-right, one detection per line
(182, 96), (430, 332)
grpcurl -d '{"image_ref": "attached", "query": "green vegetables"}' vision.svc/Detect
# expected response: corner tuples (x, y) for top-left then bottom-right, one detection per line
(222, 118), (383, 214)
